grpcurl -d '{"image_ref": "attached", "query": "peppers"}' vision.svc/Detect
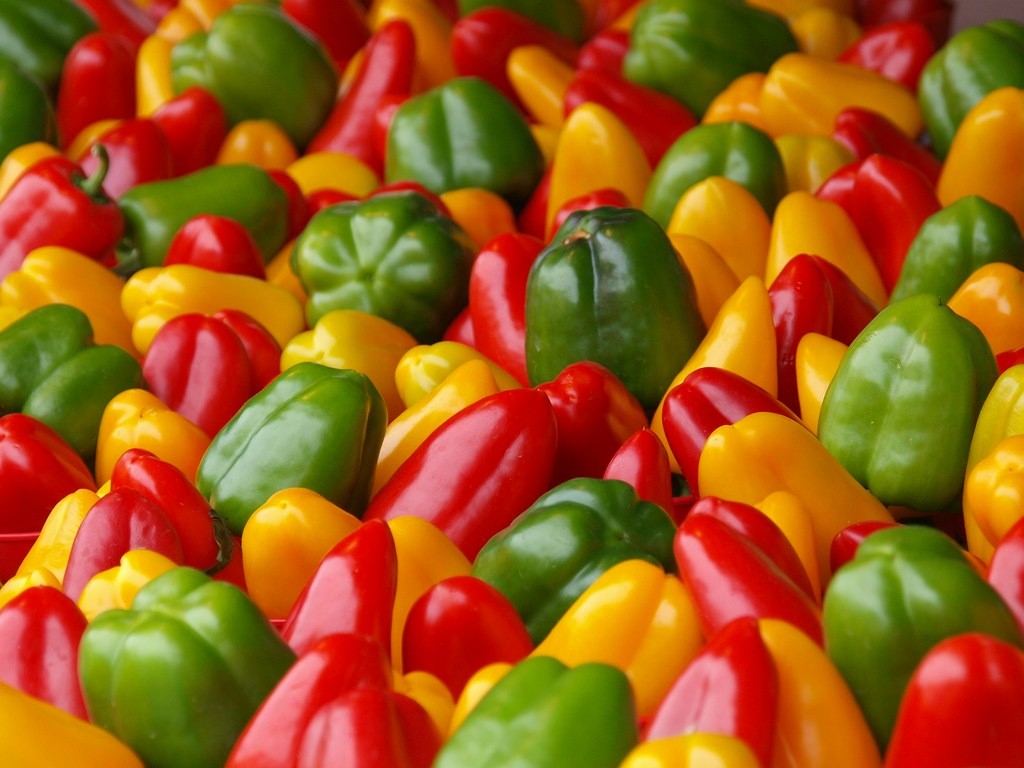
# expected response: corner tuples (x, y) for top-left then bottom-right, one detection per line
(0, 0), (1024, 768)
